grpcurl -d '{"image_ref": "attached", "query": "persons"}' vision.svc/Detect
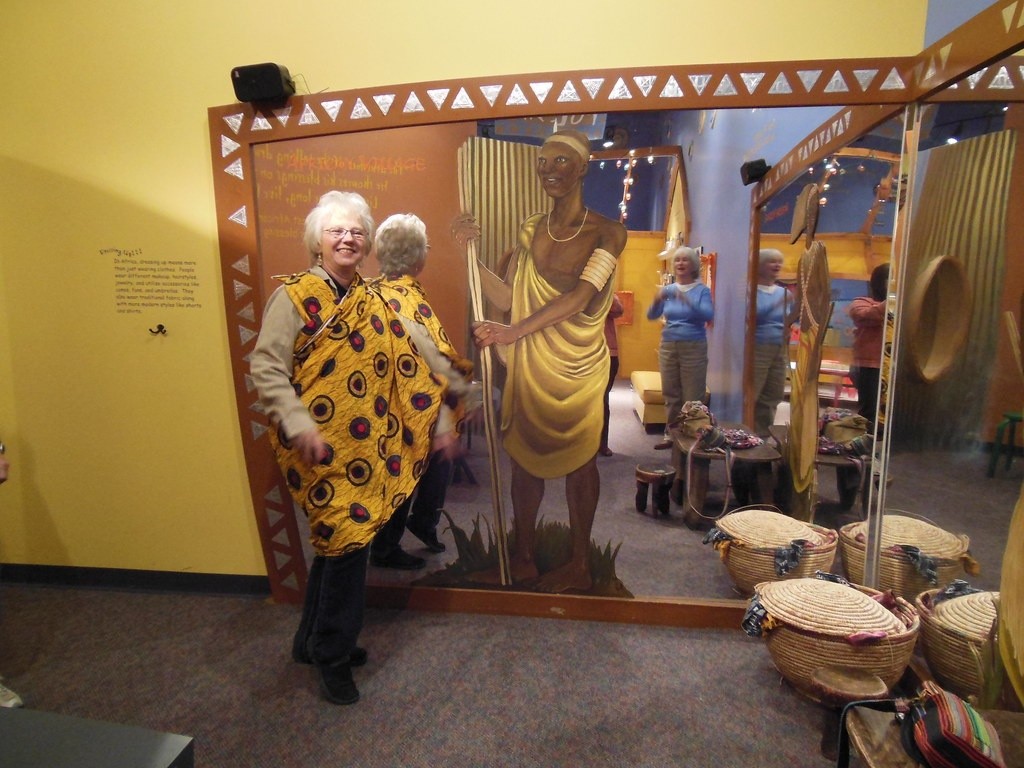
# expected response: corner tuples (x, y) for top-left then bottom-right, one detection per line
(360, 213), (478, 571)
(598, 293), (624, 456)
(0, 440), (24, 712)
(847, 264), (891, 423)
(647, 247), (714, 448)
(754, 248), (794, 437)
(248, 191), (450, 705)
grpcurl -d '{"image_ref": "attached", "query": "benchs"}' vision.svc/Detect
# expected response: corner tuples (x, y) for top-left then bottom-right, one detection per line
(630, 371), (711, 433)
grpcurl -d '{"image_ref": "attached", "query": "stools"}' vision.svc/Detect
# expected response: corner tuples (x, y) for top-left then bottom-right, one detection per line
(987, 410), (1024, 478)
(634, 463), (676, 519)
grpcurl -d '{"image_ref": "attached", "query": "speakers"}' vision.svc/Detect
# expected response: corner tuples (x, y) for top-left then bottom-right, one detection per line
(741, 159), (767, 186)
(231, 63), (294, 102)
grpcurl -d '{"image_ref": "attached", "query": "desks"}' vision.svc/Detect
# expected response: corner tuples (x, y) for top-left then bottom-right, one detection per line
(666, 423), (783, 532)
(768, 425), (869, 511)
(844, 702), (1024, 768)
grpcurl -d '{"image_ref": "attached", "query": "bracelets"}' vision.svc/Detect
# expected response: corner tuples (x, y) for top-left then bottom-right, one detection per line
(0, 442), (5, 454)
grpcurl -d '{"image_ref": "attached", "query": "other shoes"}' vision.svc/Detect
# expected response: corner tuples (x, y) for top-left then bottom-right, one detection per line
(291, 633), (368, 669)
(305, 635), (360, 705)
(598, 444), (613, 456)
(405, 514), (445, 552)
(371, 546), (426, 570)
(654, 440), (673, 449)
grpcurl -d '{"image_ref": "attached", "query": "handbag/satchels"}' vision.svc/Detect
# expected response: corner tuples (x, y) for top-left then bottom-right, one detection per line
(818, 434), (845, 455)
(694, 424), (732, 454)
(897, 679), (1006, 768)
(722, 427), (764, 449)
(663, 397), (716, 438)
(821, 406), (868, 444)
(845, 433), (874, 456)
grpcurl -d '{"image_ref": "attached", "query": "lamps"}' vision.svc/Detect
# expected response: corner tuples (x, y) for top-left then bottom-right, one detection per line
(602, 124), (615, 148)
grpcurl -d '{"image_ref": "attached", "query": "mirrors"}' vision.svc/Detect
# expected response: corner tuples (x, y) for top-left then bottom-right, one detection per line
(868, 0), (1024, 715)
(740, 103), (906, 535)
(205, 59), (911, 631)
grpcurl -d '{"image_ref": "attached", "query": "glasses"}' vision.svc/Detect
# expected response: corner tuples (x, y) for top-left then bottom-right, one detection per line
(324, 226), (368, 240)
